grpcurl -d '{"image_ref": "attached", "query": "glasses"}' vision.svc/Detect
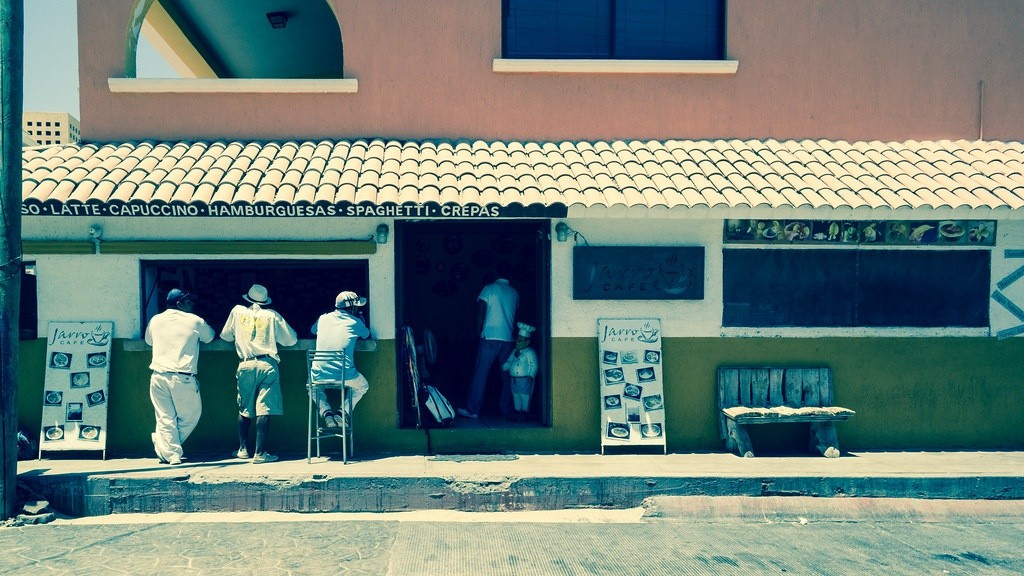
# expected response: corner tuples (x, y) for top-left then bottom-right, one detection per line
(345, 294), (360, 302)
(177, 290), (191, 301)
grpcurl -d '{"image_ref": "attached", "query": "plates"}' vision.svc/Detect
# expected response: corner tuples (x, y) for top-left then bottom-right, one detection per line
(606, 355), (616, 361)
(626, 385), (640, 397)
(646, 352), (659, 362)
(82, 426), (98, 439)
(73, 373), (88, 386)
(624, 353), (636, 361)
(639, 369), (653, 379)
(46, 393), (61, 403)
(606, 397), (619, 406)
(606, 369), (623, 383)
(46, 427), (63, 440)
(642, 424), (660, 436)
(88, 355), (106, 366)
(91, 393), (103, 403)
(610, 428), (628, 437)
(785, 221), (810, 239)
(53, 353), (68, 366)
(645, 398), (662, 409)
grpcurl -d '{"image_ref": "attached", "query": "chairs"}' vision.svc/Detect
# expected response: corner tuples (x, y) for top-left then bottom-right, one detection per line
(305, 347), (354, 465)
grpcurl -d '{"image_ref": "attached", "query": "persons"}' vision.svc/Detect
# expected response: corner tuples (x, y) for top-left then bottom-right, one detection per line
(144, 288), (215, 465)
(458, 259), (520, 419)
(502, 322), (538, 418)
(221, 285), (297, 464)
(311, 291), (370, 427)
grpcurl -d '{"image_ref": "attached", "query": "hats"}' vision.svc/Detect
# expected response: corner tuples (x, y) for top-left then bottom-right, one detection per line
(335, 291), (367, 308)
(242, 284), (272, 306)
(166, 289), (188, 308)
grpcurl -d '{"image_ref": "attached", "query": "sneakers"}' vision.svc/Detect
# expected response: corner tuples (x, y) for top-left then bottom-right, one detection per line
(231, 449), (249, 459)
(334, 411), (349, 428)
(253, 451), (279, 464)
(324, 412), (337, 428)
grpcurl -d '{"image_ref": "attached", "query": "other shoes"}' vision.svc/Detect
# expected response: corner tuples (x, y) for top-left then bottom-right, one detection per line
(170, 460), (181, 465)
(151, 432), (169, 464)
(457, 408), (478, 418)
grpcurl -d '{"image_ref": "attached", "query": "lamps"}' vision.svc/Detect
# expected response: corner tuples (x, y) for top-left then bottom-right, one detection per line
(555, 220), (568, 242)
(377, 223), (389, 244)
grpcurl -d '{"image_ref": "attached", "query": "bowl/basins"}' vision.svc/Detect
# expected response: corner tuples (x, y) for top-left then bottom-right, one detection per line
(762, 229), (777, 240)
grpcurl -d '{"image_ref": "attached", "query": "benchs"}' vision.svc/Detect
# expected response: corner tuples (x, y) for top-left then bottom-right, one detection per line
(717, 365), (856, 458)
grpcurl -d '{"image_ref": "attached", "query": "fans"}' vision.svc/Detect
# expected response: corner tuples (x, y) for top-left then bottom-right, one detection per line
(417, 329), (438, 378)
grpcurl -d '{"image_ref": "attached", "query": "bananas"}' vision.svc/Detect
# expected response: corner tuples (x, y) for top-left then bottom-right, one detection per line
(911, 225), (934, 239)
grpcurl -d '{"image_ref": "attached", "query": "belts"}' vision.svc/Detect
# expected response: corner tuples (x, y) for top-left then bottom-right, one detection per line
(170, 372), (195, 377)
(239, 354), (270, 362)
